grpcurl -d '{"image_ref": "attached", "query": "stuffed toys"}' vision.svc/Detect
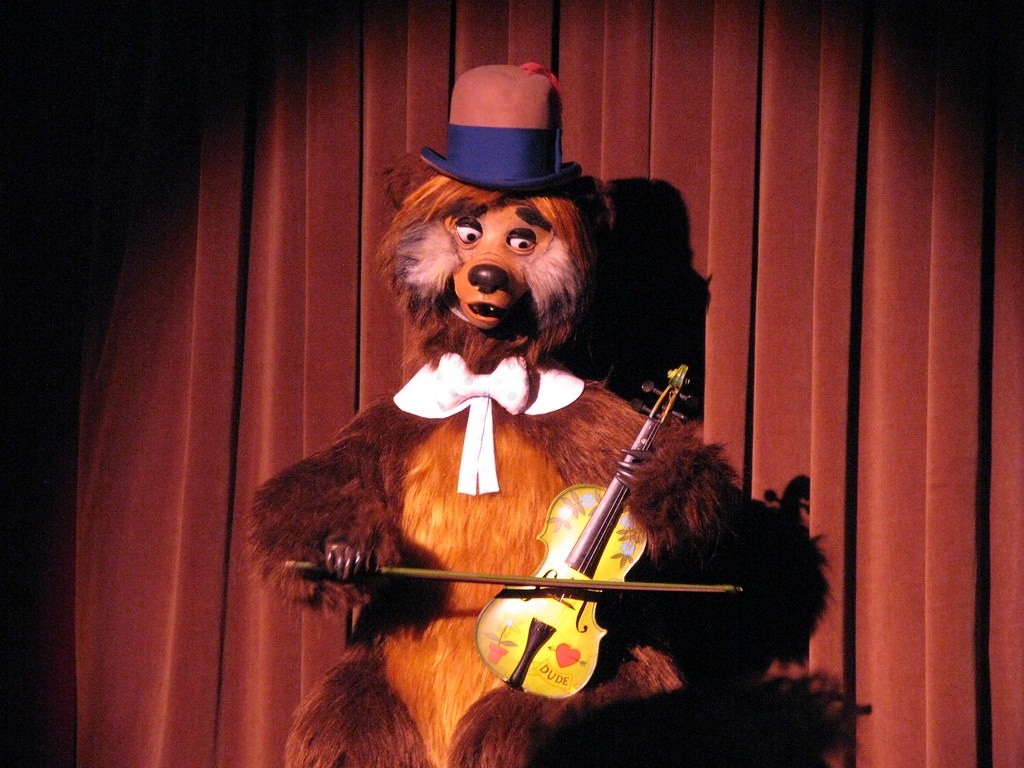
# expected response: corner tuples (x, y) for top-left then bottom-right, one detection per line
(247, 60), (753, 768)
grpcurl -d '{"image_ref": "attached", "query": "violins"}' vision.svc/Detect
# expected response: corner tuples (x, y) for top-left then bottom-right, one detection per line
(474, 365), (693, 700)
